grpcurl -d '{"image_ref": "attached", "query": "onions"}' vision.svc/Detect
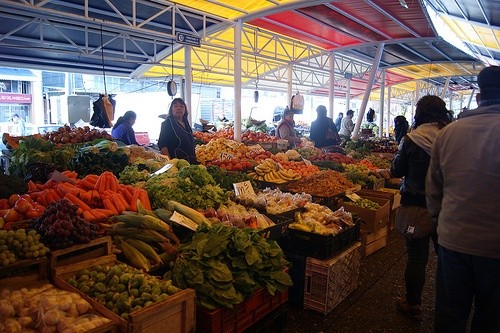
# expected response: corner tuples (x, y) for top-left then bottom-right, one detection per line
(44, 125), (117, 144)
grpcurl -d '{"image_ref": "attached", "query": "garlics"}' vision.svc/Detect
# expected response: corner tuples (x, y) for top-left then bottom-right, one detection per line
(0, 285), (112, 333)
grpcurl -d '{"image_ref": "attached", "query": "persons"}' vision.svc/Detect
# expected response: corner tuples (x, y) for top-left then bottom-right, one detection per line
(157, 98), (199, 166)
(338, 109), (356, 140)
(335, 112), (343, 132)
(425, 65), (499, 333)
(275, 109), (303, 148)
(112, 111), (146, 146)
(8, 113), (26, 136)
(310, 105), (338, 147)
(389, 96), (452, 317)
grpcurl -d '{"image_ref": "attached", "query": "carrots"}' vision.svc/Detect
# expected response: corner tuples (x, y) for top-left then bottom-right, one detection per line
(28, 171), (151, 227)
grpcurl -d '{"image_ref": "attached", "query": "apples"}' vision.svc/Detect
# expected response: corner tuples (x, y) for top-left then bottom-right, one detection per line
(229, 130), (279, 142)
(341, 163), (368, 172)
(292, 147), (321, 158)
(195, 137), (271, 171)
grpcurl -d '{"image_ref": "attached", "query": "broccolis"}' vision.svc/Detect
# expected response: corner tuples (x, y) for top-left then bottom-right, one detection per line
(274, 150), (300, 162)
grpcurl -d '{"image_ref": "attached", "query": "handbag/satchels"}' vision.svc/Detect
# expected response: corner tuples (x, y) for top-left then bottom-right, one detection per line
(394, 207), (433, 240)
(325, 119), (336, 143)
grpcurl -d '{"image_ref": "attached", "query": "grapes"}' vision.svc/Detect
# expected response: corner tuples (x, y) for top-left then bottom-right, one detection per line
(0, 229), (50, 265)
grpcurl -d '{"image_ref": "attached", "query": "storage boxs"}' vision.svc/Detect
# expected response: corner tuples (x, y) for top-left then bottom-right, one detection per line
(246, 177), (349, 212)
(54, 255), (196, 333)
(303, 241), (362, 315)
(197, 268), (290, 333)
(287, 216), (361, 260)
(337, 195), (390, 232)
(360, 225), (388, 257)
(257, 213), (294, 239)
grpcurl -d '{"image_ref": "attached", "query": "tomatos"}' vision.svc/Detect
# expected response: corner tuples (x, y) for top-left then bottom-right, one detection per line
(0, 194), (46, 230)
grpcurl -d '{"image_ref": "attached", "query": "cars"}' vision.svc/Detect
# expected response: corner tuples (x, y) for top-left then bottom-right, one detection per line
(0, 120), (65, 137)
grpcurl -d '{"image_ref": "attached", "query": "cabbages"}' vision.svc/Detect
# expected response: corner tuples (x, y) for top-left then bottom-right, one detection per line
(143, 158), (189, 173)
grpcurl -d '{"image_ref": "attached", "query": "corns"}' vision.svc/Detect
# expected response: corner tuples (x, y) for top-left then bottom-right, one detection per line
(100, 199), (211, 271)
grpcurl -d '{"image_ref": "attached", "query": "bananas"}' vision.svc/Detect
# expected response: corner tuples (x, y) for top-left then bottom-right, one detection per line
(248, 161), (301, 183)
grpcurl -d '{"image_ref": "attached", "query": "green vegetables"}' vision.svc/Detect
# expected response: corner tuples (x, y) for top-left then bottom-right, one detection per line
(120, 165), (249, 209)
(162, 220), (292, 308)
(312, 160), (345, 173)
(340, 135), (374, 159)
(346, 170), (376, 190)
(7, 138), (131, 183)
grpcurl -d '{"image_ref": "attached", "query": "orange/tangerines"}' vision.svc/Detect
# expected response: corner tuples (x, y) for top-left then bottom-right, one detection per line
(280, 161), (319, 176)
(194, 128), (233, 142)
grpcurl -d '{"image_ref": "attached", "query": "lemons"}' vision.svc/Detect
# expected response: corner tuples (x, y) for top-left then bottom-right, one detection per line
(254, 192), (331, 225)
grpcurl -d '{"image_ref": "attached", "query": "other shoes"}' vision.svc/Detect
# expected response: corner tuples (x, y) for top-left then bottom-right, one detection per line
(399, 298), (419, 316)
